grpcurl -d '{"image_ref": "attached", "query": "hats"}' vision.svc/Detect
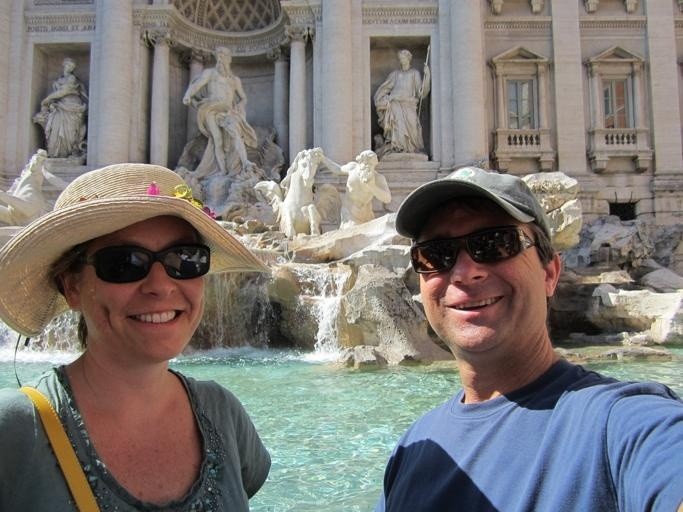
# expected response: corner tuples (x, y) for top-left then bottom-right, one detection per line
(0, 163), (271, 336)
(396, 166), (551, 239)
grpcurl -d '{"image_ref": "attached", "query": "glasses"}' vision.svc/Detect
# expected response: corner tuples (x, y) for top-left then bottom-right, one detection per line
(410, 226), (536, 274)
(81, 243), (210, 283)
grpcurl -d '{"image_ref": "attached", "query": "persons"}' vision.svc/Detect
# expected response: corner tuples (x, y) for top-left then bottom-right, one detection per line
(0, 148), (67, 226)
(371, 168), (683, 512)
(319, 150), (392, 227)
(0, 163), (272, 512)
(40, 57), (89, 158)
(183, 47), (256, 177)
(373, 49), (431, 153)
